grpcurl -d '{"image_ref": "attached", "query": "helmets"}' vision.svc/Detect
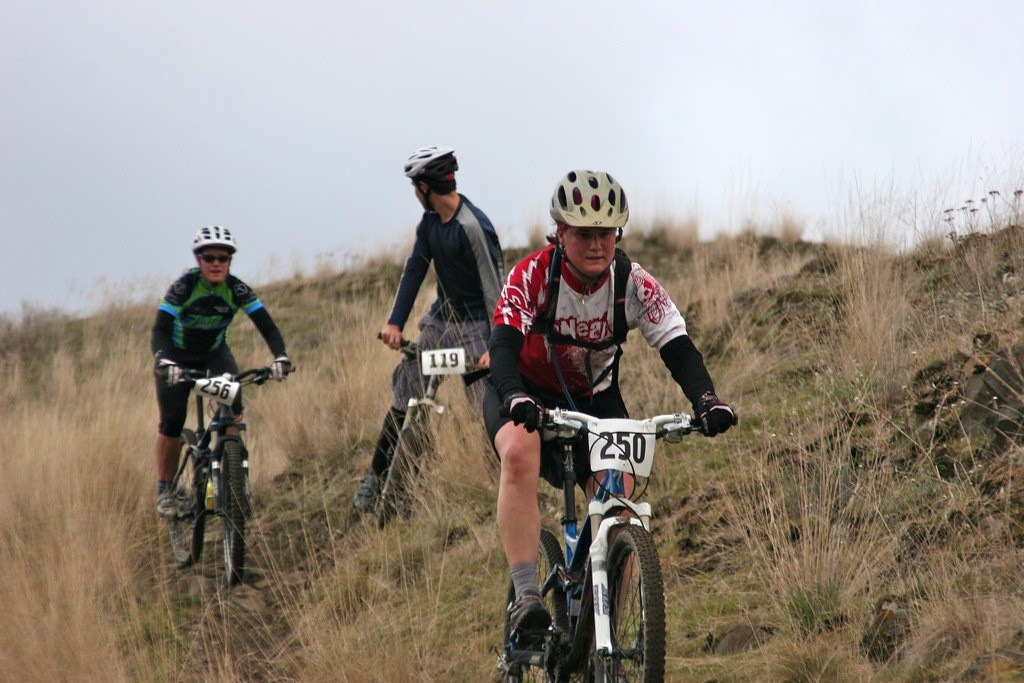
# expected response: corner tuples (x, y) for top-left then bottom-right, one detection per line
(403, 146), (458, 177)
(193, 226), (236, 257)
(550, 169), (628, 227)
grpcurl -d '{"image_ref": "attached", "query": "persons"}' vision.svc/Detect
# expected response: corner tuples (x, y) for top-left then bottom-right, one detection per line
(351, 145), (505, 510)
(482, 169), (733, 682)
(152, 225), (293, 517)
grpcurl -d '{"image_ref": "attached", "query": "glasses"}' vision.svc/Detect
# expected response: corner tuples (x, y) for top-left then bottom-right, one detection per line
(198, 253), (230, 264)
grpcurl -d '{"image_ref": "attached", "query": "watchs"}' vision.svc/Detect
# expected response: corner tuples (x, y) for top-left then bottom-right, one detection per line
(696, 392), (717, 407)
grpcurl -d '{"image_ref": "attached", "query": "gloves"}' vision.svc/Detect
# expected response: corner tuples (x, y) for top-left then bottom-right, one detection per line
(693, 392), (737, 437)
(156, 358), (183, 385)
(505, 393), (544, 433)
(269, 355), (292, 382)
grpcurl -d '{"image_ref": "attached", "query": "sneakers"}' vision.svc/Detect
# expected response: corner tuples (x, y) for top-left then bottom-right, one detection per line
(590, 662), (629, 683)
(353, 471), (380, 508)
(507, 590), (552, 653)
(157, 489), (177, 517)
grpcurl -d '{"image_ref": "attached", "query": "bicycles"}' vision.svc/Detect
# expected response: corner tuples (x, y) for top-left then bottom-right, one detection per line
(493, 402), (742, 683)
(152, 362), (295, 587)
(375, 331), (493, 533)
